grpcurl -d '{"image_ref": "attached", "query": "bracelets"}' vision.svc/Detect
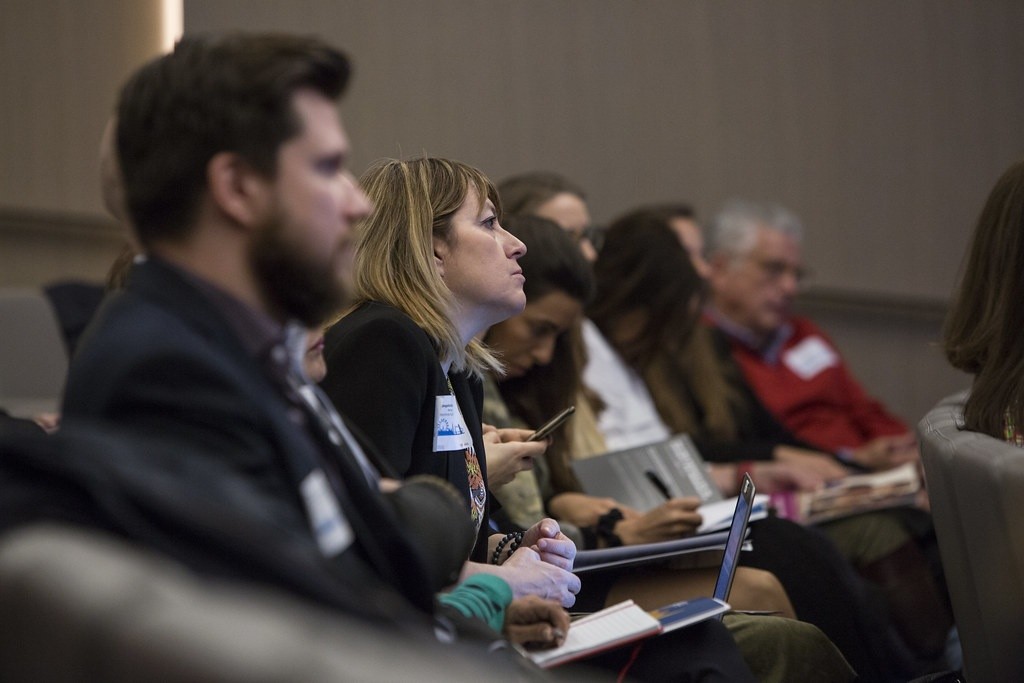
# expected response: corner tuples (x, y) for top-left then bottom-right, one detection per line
(492, 531), (525, 566)
(596, 507), (625, 547)
(734, 463), (751, 494)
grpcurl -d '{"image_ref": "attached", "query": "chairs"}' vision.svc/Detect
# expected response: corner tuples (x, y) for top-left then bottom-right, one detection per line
(917, 386), (1023, 683)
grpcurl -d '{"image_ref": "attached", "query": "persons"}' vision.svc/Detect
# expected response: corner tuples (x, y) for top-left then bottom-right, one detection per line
(289, 327), (859, 683)
(54, 34), (758, 683)
(478, 216), (703, 548)
(99, 117), (145, 293)
(587, 209), (830, 495)
(0, 408), (418, 629)
(943, 160), (1024, 451)
(659, 199), (969, 683)
(495, 173), (933, 683)
(318, 155), (794, 617)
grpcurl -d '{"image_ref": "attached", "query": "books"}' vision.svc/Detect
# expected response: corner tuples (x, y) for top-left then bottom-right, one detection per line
(806, 461), (920, 522)
(571, 524), (754, 573)
(526, 600), (664, 668)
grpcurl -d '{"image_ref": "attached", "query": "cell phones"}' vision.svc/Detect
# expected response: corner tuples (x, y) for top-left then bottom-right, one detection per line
(522, 404), (574, 464)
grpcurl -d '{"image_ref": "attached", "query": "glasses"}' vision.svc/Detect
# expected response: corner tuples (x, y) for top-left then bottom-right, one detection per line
(741, 252), (806, 284)
(560, 224), (607, 253)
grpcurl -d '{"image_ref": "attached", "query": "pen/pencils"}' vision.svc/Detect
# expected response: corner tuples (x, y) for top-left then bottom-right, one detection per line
(646, 471), (673, 501)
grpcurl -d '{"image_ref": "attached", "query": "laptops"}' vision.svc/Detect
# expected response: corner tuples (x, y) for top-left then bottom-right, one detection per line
(562, 472), (756, 640)
